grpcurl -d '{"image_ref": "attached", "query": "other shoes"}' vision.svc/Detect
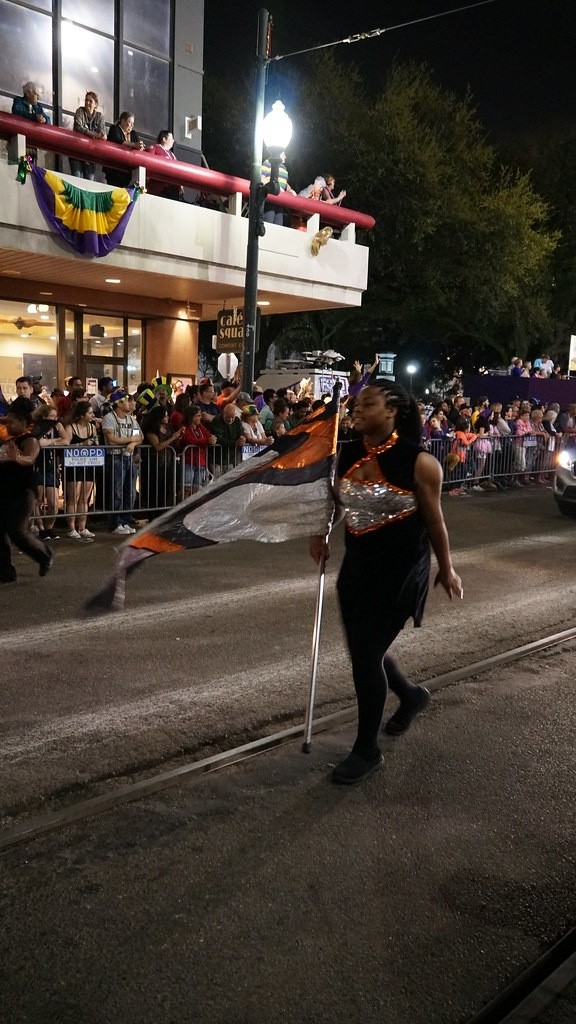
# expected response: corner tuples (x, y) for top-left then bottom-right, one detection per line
(444, 476), (551, 497)
(0, 572), (16, 584)
(385, 685), (433, 736)
(334, 740), (383, 782)
(38, 554), (55, 576)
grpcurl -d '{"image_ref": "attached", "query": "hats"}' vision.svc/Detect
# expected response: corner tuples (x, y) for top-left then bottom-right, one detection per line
(238, 392), (254, 403)
(220, 381), (236, 390)
(26, 374), (42, 384)
(459, 402), (469, 412)
(530, 397), (540, 404)
(242, 404), (260, 415)
(109, 390), (129, 404)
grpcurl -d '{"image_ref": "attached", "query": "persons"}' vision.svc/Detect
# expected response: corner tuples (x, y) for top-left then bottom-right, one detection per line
(103, 110), (148, 189)
(12, 81), (51, 166)
(146, 129), (186, 202)
(0, 354), (576, 584)
(260, 148), (346, 240)
(310, 377), (464, 782)
(69, 91), (108, 181)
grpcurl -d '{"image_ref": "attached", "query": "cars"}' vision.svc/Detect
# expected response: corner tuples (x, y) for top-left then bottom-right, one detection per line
(553, 446), (576, 515)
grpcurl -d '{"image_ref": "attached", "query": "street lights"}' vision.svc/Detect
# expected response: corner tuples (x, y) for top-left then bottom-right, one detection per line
(233, 1), (294, 397)
(407, 365), (417, 398)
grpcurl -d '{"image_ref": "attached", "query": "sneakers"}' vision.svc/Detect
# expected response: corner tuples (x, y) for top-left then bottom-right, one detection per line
(78, 529), (95, 538)
(49, 528), (59, 540)
(112, 524), (130, 534)
(68, 529), (82, 540)
(38, 529), (51, 540)
(124, 524), (136, 534)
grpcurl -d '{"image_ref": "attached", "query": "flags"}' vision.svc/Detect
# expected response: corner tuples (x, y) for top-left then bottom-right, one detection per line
(106, 398), (338, 610)
